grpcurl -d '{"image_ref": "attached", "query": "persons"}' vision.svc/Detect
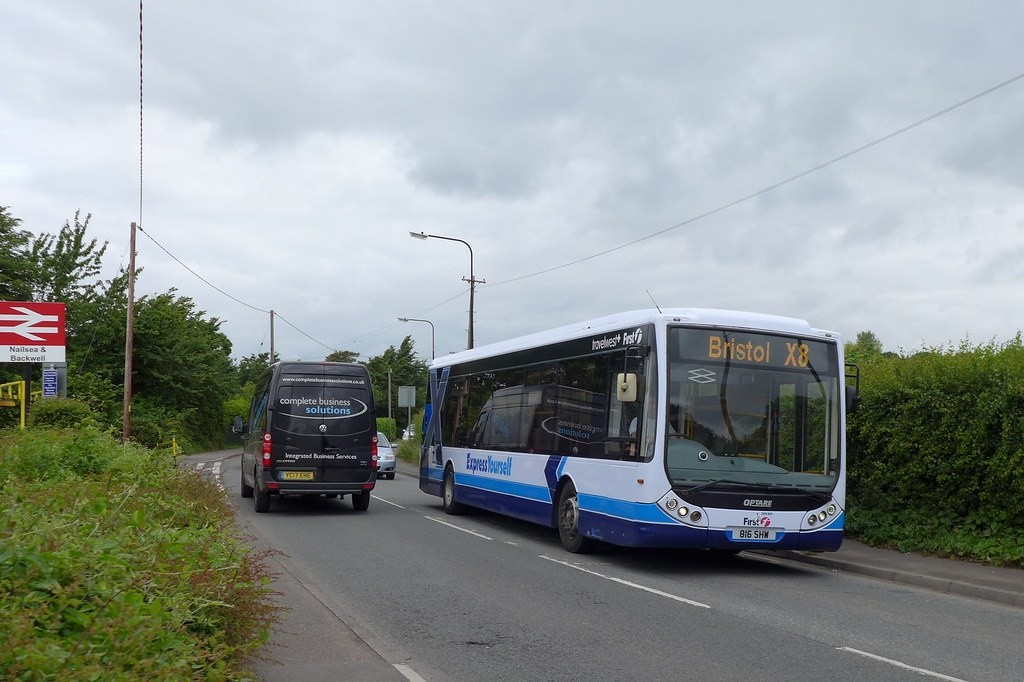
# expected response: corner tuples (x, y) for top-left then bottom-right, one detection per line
(630, 392), (680, 456)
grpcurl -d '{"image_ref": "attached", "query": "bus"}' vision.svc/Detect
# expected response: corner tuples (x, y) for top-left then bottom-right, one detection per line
(419, 308), (861, 553)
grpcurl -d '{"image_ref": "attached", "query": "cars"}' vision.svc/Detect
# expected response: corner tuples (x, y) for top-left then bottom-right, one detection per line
(377, 432), (400, 482)
(402, 423), (415, 442)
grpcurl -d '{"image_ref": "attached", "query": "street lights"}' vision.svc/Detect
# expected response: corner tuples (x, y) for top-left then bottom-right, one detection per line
(396, 317), (435, 360)
(409, 230), (477, 351)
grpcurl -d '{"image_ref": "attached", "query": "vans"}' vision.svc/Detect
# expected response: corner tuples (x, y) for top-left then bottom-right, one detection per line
(234, 361), (379, 514)
